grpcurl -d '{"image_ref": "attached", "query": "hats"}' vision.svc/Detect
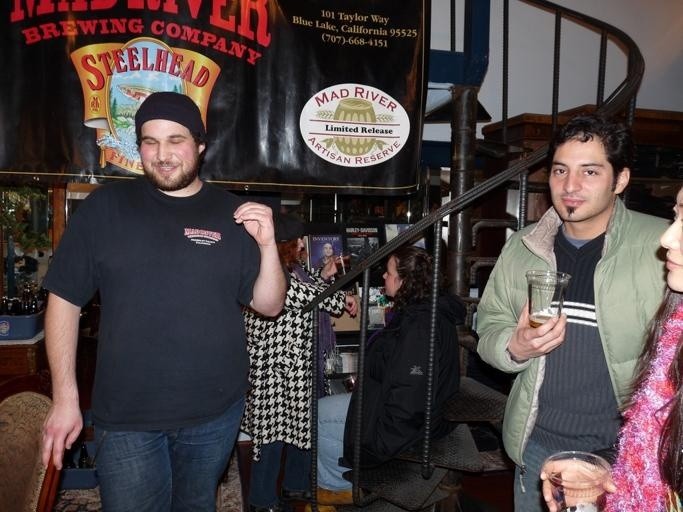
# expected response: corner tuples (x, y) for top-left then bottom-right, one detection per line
(136, 92), (207, 139)
(272, 213), (305, 244)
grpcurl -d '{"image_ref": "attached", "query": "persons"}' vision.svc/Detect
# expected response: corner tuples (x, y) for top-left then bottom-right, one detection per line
(475, 109), (672, 512)
(37, 91), (288, 512)
(240, 214), (358, 512)
(338, 246), (461, 502)
(536, 184), (683, 512)
(318, 242), (336, 266)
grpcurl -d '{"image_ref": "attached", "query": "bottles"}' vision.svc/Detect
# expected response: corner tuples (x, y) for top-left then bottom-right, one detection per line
(78, 444), (91, 467)
(62, 454), (76, 468)
(0, 281), (47, 315)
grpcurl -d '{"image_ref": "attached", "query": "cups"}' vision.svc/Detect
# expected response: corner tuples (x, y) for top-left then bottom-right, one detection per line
(331, 250), (351, 277)
(540, 449), (612, 511)
(525, 270), (573, 328)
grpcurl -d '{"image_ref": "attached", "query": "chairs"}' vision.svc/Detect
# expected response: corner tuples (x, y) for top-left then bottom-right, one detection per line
(1, 371), (69, 511)
